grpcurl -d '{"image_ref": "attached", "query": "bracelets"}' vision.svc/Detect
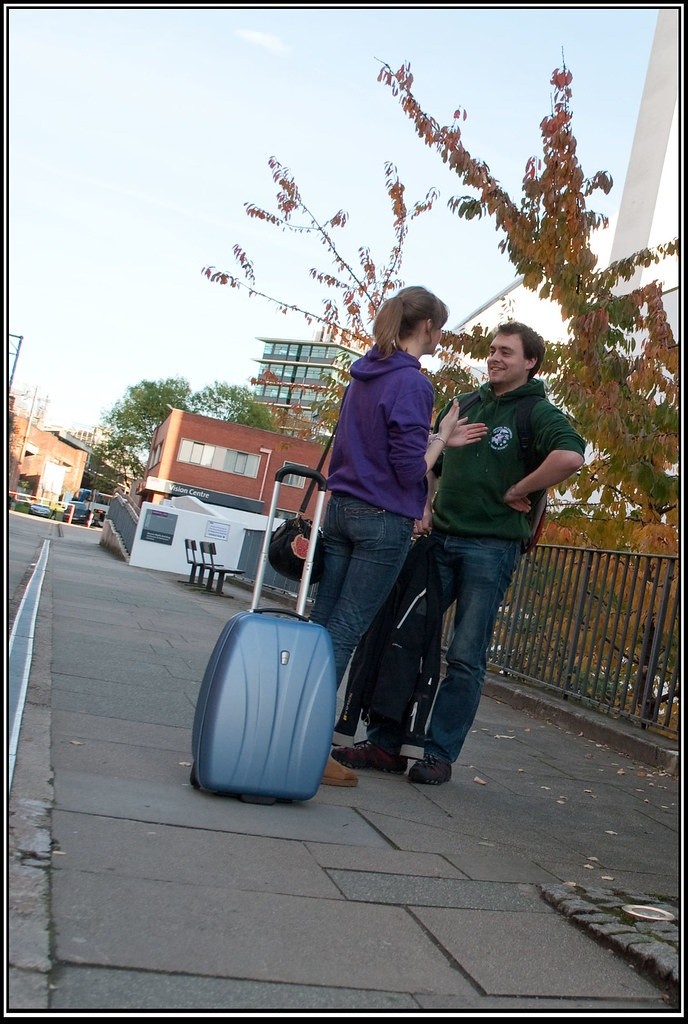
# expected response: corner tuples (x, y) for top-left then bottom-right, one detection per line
(435, 437), (446, 444)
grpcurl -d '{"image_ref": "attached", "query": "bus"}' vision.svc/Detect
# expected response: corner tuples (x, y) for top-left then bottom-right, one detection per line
(79, 489), (115, 522)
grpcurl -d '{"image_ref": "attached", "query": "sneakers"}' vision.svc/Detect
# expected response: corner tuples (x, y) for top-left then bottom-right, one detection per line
(409, 754), (452, 785)
(321, 756), (359, 787)
(331, 743), (408, 775)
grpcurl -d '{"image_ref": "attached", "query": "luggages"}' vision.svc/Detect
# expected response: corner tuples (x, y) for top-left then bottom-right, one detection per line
(189, 465), (337, 805)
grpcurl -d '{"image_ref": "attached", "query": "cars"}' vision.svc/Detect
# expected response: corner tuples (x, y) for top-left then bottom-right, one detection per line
(30, 503), (51, 518)
(61, 501), (90, 524)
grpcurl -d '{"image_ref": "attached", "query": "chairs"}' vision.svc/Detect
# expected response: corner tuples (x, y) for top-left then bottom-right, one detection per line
(178, 539), (224, 589)
(199, 541), (246, 598)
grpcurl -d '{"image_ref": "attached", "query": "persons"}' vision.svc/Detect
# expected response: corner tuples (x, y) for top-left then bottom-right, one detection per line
(309, 287), (488, 786)
(331, 321), (587, 788)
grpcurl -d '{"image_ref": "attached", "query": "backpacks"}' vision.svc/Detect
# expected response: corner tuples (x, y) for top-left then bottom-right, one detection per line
(458, 391), (547, 554)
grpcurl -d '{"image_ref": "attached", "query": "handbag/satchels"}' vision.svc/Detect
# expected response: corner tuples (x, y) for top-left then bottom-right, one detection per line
(269, 516), (324, 585)
